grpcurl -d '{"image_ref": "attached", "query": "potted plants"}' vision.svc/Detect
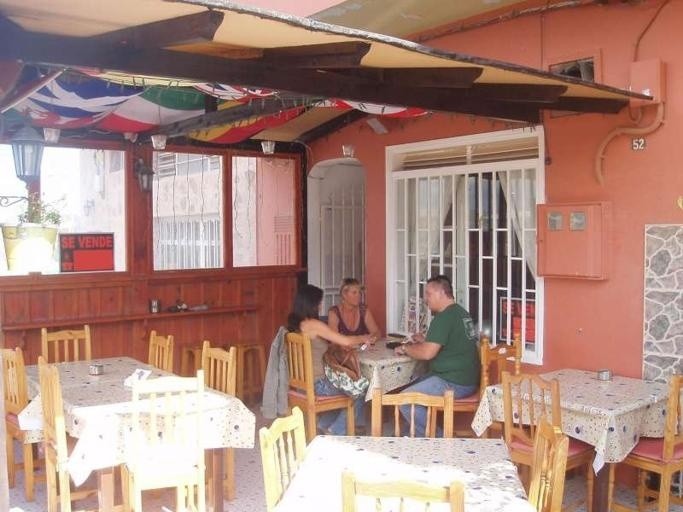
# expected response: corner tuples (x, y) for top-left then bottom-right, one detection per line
(0, 188), (71, 276)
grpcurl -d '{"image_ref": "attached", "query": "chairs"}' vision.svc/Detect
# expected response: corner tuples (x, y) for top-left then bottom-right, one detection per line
(38, 322), (93, 365)
(603, 369), (682, 512)
(115, 365), (211, 511)
(33, 355), (126, 511)
(257, 404), (307, 512)
(0, 347), (42, 511)
(338, 468), (465, 511)
(479, 330), (530, 484)
(146, 328), (174, 375)
(276, 330), (356, 452)
(359, 337), (486, 439)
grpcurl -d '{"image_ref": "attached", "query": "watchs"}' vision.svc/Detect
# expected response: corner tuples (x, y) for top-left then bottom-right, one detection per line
(401, 344), (408, 356)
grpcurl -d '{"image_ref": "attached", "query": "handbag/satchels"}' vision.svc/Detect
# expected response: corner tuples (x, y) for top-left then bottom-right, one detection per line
(322, 346), (369, 395)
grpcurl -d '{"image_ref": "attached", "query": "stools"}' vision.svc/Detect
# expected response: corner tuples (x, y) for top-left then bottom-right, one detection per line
(227, 339), (267, 406)
(181, 345), (201, 378)
(199, 341), (238, 503)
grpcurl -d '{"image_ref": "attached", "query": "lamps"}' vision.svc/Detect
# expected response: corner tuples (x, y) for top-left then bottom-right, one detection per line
(0, 118), (45, 189)
(41, 81), (63, 145)
(148, 90), (169, 150)
(260, 140), (275, 155)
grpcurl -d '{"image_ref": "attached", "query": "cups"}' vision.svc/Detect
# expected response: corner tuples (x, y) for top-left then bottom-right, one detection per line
(149, 298), (160, 313)
(597, 370), (609, 380)
(88, 363), (103, 375)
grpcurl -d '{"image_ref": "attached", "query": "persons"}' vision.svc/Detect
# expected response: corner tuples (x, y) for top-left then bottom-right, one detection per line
(287, 285), (375, 436)
(392, 275), (481, 439)
(325, 276), (382, 436)
